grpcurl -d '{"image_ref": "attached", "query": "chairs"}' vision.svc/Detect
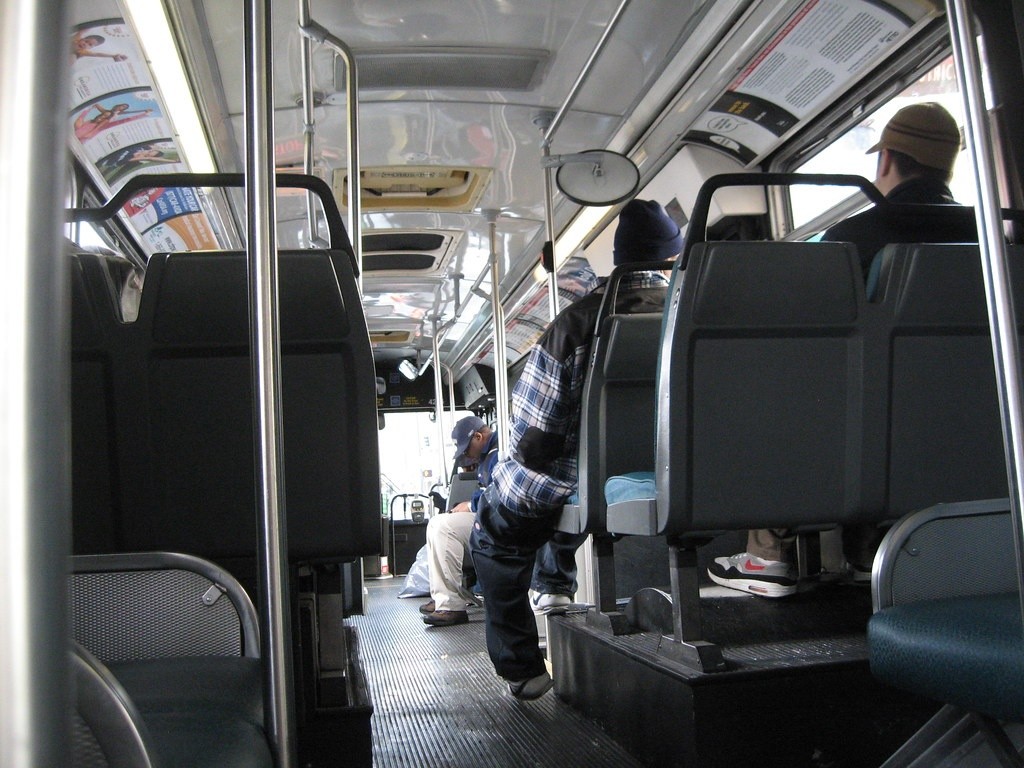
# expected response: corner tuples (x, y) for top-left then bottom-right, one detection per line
(64, 170), (1024, 768)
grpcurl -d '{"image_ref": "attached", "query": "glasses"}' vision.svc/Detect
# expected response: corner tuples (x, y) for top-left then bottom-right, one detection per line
(463, 434), (474, 454)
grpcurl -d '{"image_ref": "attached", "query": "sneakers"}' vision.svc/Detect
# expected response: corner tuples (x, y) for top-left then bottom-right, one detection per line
(508, 670), (556, 699)
(530, 590), (572, 615)
(706, 551), (798, 599)
(420, 598), (440, 614)
(423, 609), (468, 626)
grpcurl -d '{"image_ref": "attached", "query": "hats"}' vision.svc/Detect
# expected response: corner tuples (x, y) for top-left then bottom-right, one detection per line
(451, 416), (486, 459)
(866, 102), (960, 171)
(613, 198), (685, 266)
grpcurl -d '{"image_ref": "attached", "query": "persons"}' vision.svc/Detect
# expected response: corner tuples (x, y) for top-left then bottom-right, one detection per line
(100, 144), (163, 168)
(419, 415), (499, 626)
(467, 198), (684, 701)
(707, 102), (1010, 596)
(72, 28), (128, 62)
(75, 103), (152, 144)
